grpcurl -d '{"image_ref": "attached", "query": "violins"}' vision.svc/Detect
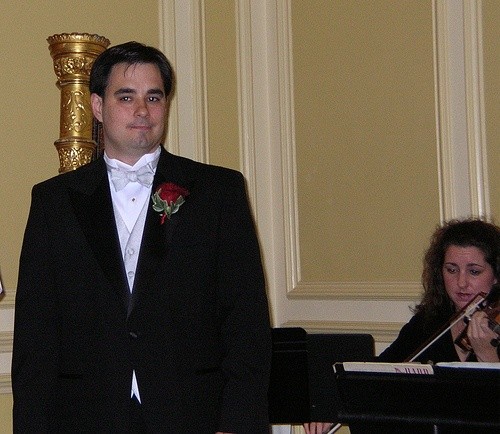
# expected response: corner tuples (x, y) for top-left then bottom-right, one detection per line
(454, 305), (500, 349)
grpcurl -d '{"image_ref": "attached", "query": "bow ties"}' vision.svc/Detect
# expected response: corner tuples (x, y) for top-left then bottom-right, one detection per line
(110, 169), (154, 192)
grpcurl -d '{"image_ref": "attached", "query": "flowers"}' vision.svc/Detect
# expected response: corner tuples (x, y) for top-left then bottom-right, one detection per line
(151, 183), (190, 227)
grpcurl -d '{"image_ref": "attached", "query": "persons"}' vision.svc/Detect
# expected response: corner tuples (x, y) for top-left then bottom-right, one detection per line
(304, 214), (500, 434)
(11, 41), (272, 434)
